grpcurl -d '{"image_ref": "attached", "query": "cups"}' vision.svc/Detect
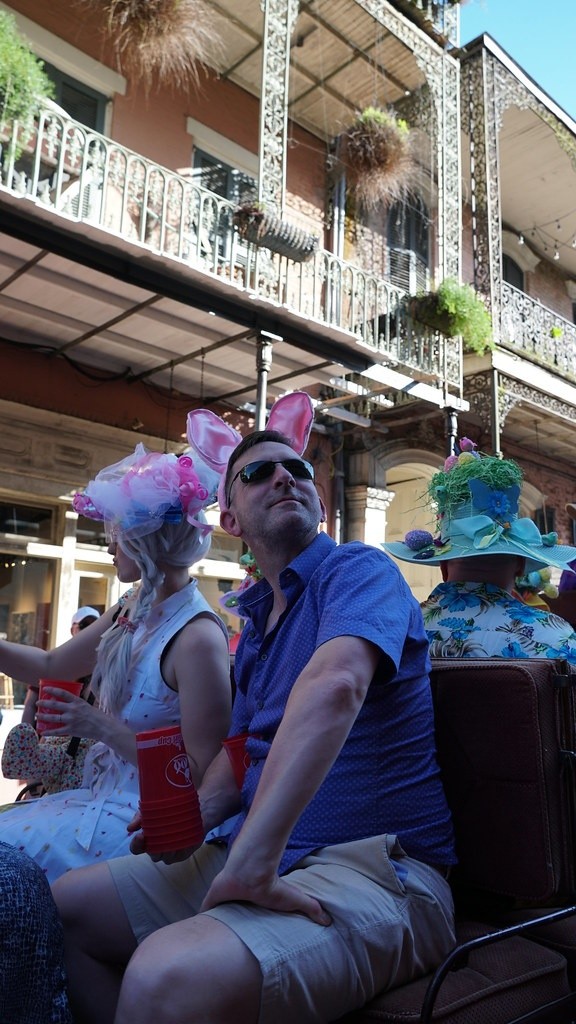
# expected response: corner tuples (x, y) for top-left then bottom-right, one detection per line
(222, 733), (250, 791)
(36, 679), (84, 736)
(135, 726), (204, 853)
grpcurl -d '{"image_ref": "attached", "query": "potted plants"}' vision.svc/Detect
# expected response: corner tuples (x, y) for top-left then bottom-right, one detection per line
(338, 106), (422, 217)
(105, 0), (230, 97)
(231, 196), (320, 265)
(408, 278), (497, 358)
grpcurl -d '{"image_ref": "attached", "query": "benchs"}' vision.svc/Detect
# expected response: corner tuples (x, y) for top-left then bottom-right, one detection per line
(11, 657), (576, 1024)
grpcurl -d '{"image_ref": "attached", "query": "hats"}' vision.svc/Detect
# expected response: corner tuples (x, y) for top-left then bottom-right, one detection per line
(65, 447), (230, 543)
(380, 444), (576, 573)
(71, 606), (101, 626)
(506, 518), (576, 565)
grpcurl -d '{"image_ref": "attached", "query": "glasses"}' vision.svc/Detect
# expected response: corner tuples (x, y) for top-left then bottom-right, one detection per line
(227, 458), (315, 510)
(77, 621), (89, 631)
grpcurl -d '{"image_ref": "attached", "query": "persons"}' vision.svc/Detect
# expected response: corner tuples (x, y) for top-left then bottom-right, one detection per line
(0, 441), (231, 885)
(384, 436), (576, 910)
(50, 432), (459, 1024)
(0, 840), (73, 1024)
(19, 604), (101, 801)
